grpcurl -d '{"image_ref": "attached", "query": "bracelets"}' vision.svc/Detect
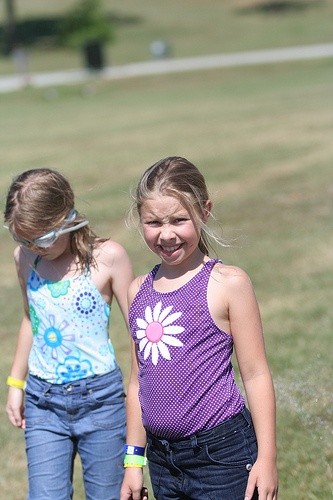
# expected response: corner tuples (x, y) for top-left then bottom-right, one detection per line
(120, 444), (147, 468)
(5, 375), (26, 392)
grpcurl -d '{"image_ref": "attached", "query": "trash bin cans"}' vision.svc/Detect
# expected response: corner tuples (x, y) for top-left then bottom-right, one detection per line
(82, 43), (103, 71)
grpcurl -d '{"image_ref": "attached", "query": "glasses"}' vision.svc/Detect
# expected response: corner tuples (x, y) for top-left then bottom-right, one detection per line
(12, 211), (77, 251)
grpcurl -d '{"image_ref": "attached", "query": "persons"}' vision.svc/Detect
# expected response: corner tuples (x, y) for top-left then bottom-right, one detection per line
(2, 168), (134, 500)
(118, 156), (277, 500)
